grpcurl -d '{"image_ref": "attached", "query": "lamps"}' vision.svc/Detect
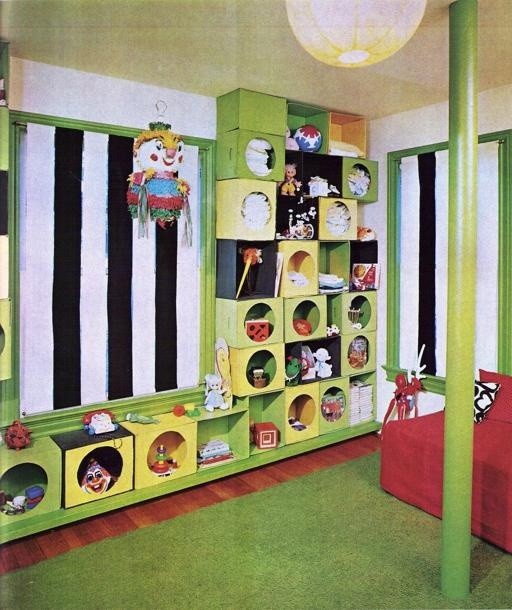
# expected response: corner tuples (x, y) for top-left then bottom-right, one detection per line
(287, 0), (428, 67)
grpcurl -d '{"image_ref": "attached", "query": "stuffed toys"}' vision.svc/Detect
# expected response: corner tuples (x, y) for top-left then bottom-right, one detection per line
(285, 348), (332, 387)
(126, 110), (194, 250)
(204, 375), (229, 411)
(278, 165), (302, 196)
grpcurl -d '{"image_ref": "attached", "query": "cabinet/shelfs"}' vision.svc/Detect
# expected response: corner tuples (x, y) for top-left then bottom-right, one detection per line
(0, 401), (252, 540)
(213, 86), (383, 462)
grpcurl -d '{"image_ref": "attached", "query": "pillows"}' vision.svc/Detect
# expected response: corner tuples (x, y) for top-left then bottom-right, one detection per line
(473, 368), (511, 425)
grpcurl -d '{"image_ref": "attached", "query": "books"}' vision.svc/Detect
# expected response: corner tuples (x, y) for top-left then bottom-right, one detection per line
(196, 439), (234, 468)
(350, 379), (373, 424)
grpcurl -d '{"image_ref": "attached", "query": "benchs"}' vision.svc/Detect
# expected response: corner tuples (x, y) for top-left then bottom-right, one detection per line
(380, 407), (510, 560)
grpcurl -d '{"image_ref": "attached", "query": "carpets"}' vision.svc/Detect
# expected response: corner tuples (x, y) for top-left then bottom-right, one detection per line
(0, 450), (512, 608)
